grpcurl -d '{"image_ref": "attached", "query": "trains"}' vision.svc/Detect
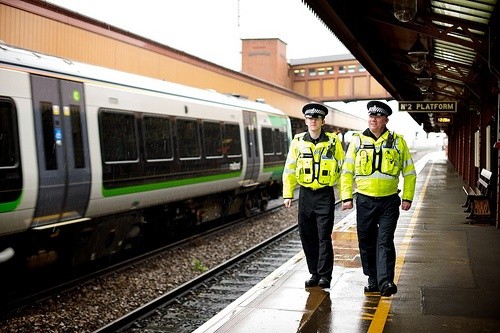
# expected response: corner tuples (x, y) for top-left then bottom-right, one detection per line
(1, 38), (293, 291)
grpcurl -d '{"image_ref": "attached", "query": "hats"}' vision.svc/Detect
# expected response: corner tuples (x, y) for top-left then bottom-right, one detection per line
(367, 101), (392, 117)
(302, 103), (328, 118)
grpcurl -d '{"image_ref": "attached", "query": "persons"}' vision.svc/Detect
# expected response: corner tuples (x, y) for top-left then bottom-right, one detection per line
(340, 101), (416, 297)
(282, 103), (344, 288)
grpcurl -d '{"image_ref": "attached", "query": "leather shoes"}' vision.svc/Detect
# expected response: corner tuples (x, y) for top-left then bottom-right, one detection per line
(318, 275), (330, 287)
(364, 282), (377, 291)
(305, 273), (321, 286)
(380, 281), (397, 298)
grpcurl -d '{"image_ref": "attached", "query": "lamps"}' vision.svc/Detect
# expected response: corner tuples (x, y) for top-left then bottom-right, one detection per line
(416, 70), (432, 91)
(393, 0), (418, 22)
(408, 33), (429, 71)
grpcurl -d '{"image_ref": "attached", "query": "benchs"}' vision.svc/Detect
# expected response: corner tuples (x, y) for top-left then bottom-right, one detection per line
(462, 168), (494, 219)
